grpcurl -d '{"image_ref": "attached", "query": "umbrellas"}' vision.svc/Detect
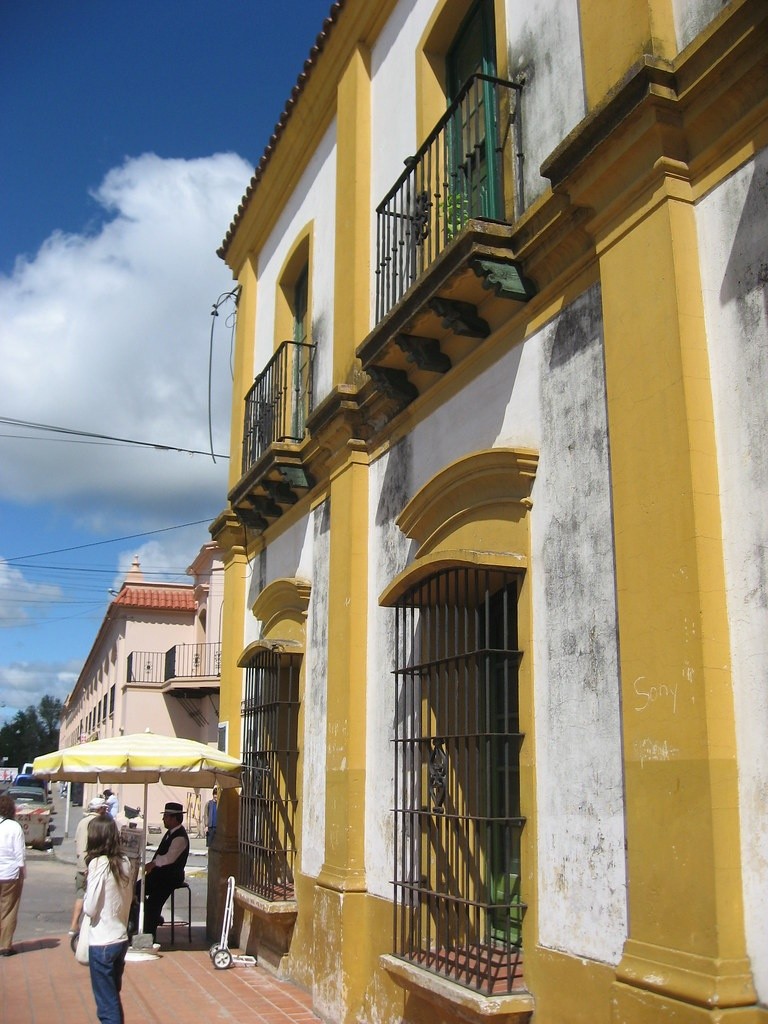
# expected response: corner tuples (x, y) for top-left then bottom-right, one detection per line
(32, 725), (244, 934)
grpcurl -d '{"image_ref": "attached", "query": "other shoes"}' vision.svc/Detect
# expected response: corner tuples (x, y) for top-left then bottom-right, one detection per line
(3, 949), (16, 955)
(130, 920), (139, 934)
(69, 929), (79, 936)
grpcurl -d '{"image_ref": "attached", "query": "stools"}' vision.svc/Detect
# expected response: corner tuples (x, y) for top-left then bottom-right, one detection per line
(156, 883), (191, 945)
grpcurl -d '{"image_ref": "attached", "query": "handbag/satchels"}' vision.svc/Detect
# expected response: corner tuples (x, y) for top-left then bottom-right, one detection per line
(75, 915), (92, 966)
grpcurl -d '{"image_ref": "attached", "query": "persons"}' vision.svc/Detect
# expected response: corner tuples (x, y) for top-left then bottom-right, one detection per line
(82, 814), (133, 1024)
(204, 787), (217, 847)
(68, 789), (190, 945)
(0, 794), (26, 958)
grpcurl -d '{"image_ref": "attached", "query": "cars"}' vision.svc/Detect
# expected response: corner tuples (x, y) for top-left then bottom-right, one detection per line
(4, 762), (53, 804)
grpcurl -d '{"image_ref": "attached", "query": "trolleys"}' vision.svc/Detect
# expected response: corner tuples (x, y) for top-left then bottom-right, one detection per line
(208, 875), (261, 969)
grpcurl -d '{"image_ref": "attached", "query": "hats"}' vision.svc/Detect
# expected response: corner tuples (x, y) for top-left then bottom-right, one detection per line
(89, 798), (109, 811)
(160, 803), (187, 813)
